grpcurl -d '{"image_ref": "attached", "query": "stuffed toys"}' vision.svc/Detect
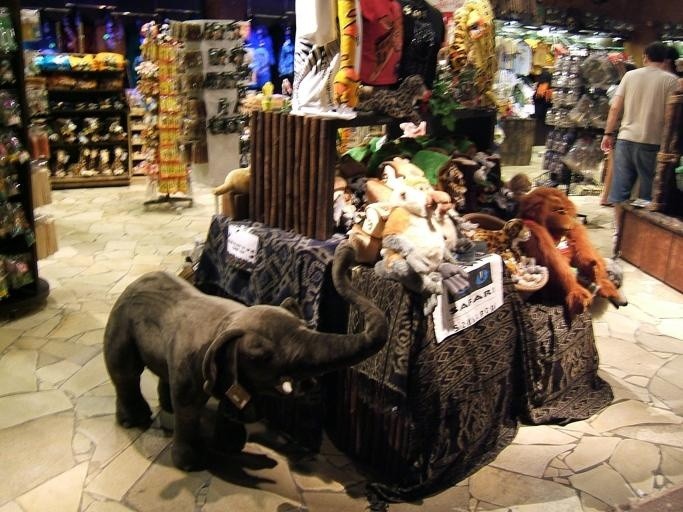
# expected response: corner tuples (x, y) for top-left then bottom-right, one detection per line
(101, 239), (387, 474)
(36, 53), (129, 180)
(346, 145), (628, 318)
(346, 145), (630, 319)
(355, 73), (433, 128)
(331, 0), (364, 107)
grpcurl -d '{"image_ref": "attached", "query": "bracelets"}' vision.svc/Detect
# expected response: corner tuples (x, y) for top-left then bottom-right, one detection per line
(601, 131), (615, 137)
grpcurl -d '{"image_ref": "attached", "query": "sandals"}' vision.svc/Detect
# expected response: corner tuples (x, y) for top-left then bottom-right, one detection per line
(601, 202), (616, 208)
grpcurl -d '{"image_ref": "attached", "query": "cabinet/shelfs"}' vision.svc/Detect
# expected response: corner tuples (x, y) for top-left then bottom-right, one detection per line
(0, 0), (683, 484)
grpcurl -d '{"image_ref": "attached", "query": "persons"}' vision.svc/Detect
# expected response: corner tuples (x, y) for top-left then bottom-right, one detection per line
(599, 42), (679, 207)
